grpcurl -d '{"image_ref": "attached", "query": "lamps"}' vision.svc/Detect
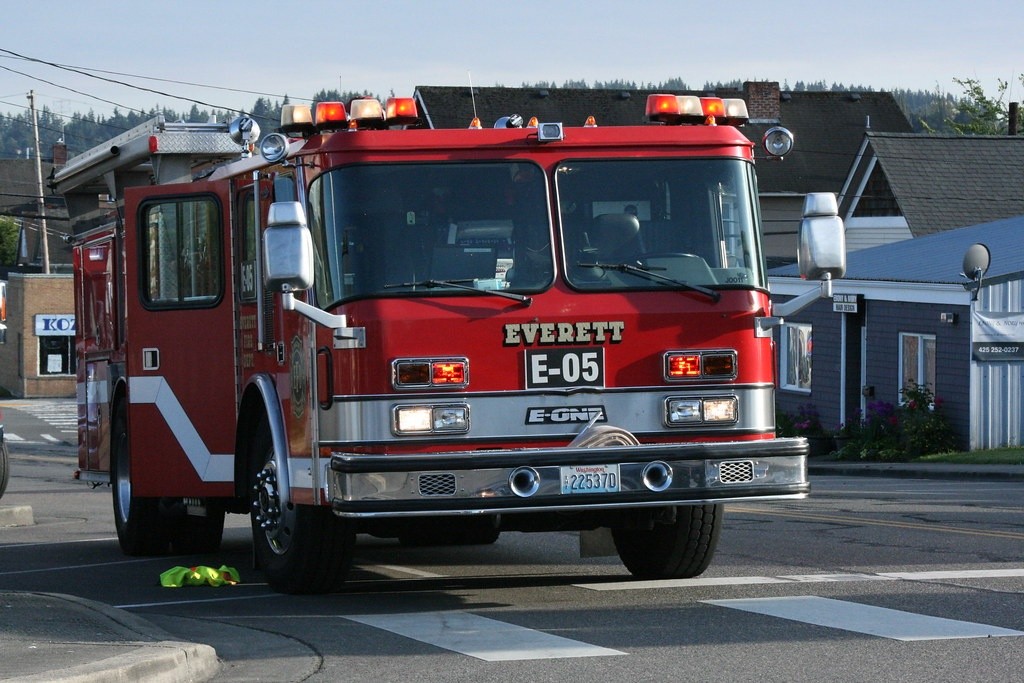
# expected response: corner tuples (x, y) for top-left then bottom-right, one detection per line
(941, 313), (958, 322)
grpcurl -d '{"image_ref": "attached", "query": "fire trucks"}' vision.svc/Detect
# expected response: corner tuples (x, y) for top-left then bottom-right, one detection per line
(46, 81), (846, 581)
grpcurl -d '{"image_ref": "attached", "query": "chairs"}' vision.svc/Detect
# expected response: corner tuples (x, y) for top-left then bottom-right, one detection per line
(362, 216), (498, 286)
(585, 213), (646, 269)
